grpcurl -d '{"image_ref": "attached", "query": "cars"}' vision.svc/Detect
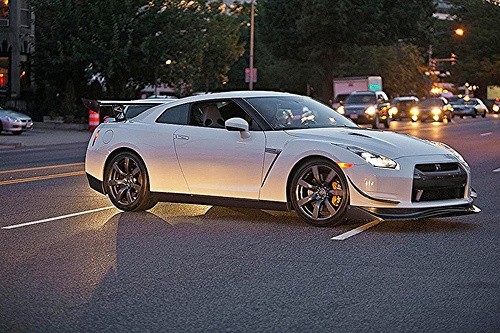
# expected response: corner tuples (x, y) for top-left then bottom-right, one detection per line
(80, 88), (481, 228)
(0, 104), (34, 135)
(411, 96), (454, 123)
(449, 95), (500, 118)
(389, 96), (419, 120)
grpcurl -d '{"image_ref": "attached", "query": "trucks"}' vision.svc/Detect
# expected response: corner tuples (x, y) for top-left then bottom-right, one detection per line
(329, 76), (382, 111)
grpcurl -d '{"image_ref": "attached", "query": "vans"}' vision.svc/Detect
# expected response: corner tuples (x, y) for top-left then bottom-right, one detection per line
(140, 88), (177, 99)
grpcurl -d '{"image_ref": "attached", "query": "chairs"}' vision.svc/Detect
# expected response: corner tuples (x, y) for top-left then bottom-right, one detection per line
(203, 106), (225, 128)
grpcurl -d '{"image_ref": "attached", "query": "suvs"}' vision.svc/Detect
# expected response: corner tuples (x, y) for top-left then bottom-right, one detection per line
(337, 90), (392, 128)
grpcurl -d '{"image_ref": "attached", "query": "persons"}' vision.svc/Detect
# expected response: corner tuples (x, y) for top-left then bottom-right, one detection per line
(203, 100), (277, 130)
(336, 100), (346, 117)
(327, 99), (335, 111)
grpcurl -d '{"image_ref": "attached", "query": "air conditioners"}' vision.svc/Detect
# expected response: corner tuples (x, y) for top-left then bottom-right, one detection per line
(0, 19), (9, 26)
